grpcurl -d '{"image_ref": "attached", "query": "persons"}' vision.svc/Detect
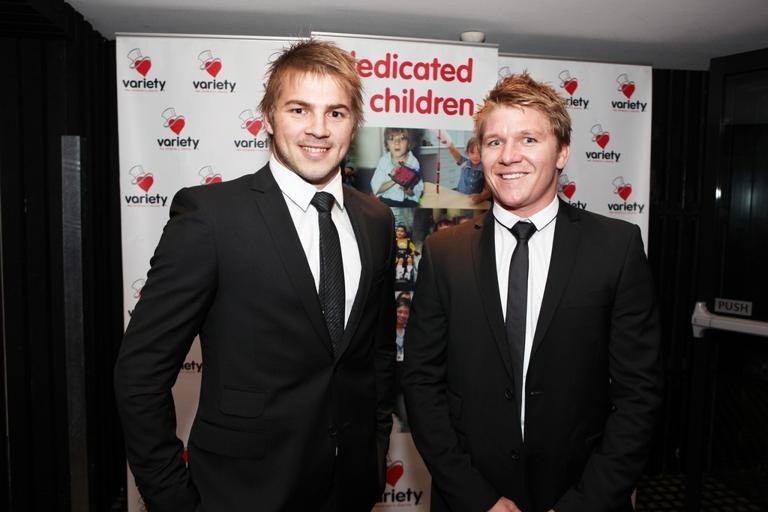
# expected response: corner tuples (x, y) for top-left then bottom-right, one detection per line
(435, 129), (492, 206)
(115, 32), (398, 512)
(391, 218), (476, 359)
(369, 129), (425, 208)
(400, 69), (663, 512)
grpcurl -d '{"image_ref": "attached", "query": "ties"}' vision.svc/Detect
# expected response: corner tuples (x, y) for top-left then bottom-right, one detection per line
(310, 191), (346, 360)
(505, 221), (537, 418)
